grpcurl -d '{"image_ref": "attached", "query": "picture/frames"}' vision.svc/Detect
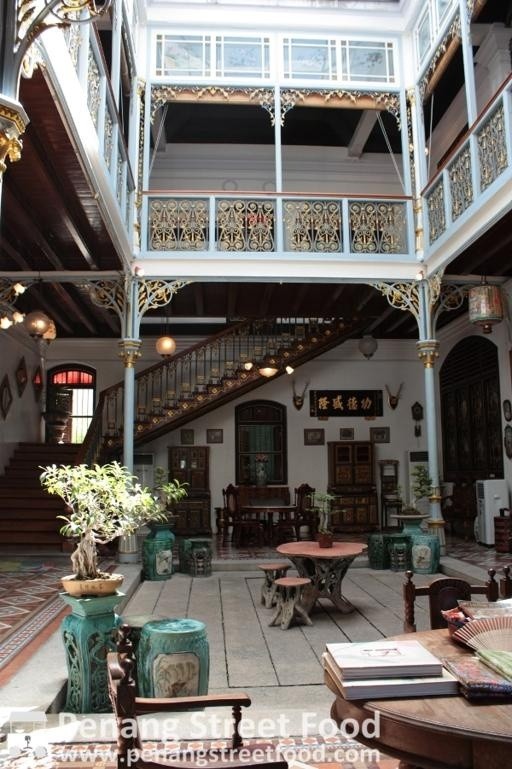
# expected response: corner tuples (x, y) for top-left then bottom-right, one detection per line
(369, 427), (391, 444)
(340, 428), (354, 440)
(206, 429), (223, 443)
(180, 429), (194, 444)
(304, 428), (325, 446)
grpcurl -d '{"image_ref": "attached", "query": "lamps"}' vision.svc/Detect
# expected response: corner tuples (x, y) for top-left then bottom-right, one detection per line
(42, 319), (56, 348)
(244, 337), (295, 378)
(358, 331), (378, 361)
(24, 273), (49, 343)
(155, 304), (176, 357)
(468, 274), (505, 334)
(0, 283), (27, 329)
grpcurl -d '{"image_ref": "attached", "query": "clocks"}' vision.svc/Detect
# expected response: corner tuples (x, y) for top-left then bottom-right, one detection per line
(411, 401), (425, 448)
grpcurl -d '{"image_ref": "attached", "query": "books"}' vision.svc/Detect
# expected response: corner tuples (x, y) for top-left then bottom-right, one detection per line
(323, 639), (444, 679)
(319, 649), (459, 701)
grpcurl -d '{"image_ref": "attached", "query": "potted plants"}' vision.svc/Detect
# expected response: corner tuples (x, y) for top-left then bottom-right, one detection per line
(38, 460), (190, 598)
(303, 491), (347, 549)
(40, 383), (72, 444)
(389, 464), (434, 543)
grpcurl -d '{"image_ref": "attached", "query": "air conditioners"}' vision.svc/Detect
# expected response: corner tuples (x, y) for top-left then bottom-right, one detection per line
(407, 446), (430, 513)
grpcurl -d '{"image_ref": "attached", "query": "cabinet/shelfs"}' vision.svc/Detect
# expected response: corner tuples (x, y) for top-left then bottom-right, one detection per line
(166, 446), (213, 539)
(327, 441), (378, 534)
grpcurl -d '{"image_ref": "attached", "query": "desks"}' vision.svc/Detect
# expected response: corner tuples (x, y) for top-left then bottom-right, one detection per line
(276, 541), (369, 613)
(240, 505), (301, 546)
(332, 629), (512, 769)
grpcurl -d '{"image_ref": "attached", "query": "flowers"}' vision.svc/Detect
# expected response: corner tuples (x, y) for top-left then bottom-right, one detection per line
(255, 454), (269, 463)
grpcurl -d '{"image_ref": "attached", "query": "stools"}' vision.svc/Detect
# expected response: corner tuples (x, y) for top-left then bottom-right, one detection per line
(136, 618), (209, 715)
(140, 539), (174, 581)
(259, 562), (292, 609)
(267, 577), (314, 630)
(178, 538), (198, 573)
(236, 520), (264, 548)
(411, 533), (440, 574)
(368, 534), (391, 570)
(269, 521), (297, 548)
(189, 538), (213, 577)
(386, 533), (412, 571)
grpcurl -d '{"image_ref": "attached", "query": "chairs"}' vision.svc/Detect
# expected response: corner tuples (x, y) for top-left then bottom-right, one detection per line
(499, 565), (512, 600)
(402, 568), (499, 634)
(106, 622), (289, 769)
(214, 483), (243, 542)
(292, 483), (321, 542)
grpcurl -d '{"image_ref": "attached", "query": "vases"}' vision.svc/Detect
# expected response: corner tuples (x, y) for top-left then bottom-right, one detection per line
(256, 462), (268, 485)
